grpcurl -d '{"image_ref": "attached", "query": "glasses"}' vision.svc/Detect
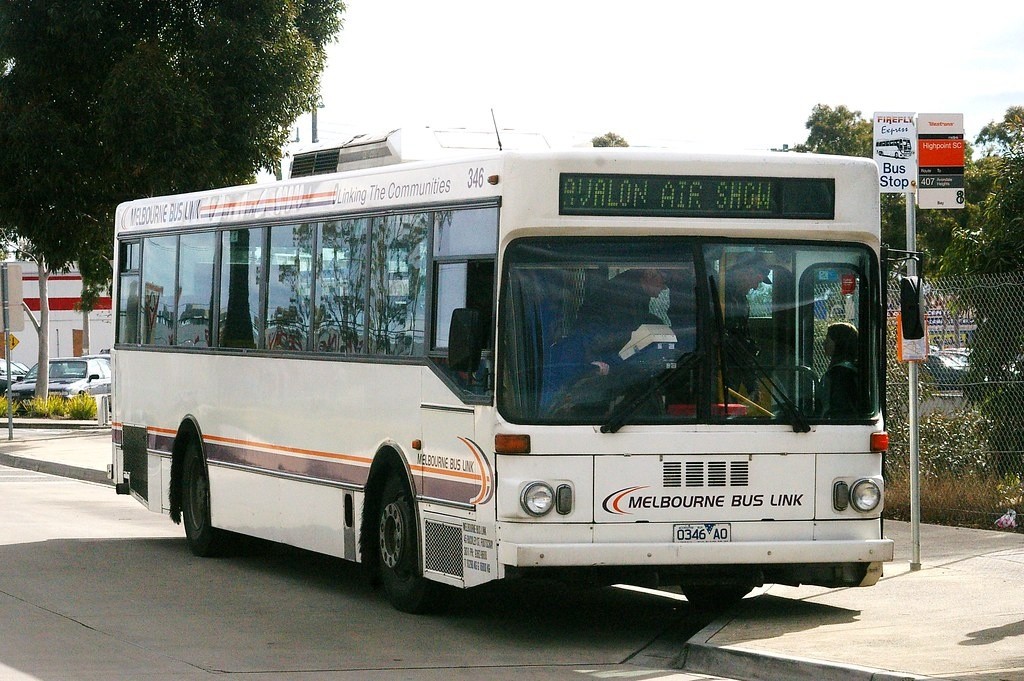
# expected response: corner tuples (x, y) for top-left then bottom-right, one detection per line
(660, 271), (669, 285)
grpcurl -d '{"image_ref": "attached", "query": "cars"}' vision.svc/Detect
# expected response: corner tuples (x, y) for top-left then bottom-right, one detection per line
(925, 344), (1019, 391)
(0, 354), (112, 411)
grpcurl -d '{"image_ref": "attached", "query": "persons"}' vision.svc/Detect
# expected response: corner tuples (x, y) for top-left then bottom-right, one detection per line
(813, 325), (874, 421)
(571, 262), (774, 420)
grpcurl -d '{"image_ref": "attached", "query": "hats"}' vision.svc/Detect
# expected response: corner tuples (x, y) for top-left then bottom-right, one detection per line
(737, 253), (771, 285)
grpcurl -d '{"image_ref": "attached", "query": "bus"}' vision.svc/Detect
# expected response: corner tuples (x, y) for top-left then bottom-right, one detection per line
(875, 137), (912, 159)
(110, 126), (928, 616)
